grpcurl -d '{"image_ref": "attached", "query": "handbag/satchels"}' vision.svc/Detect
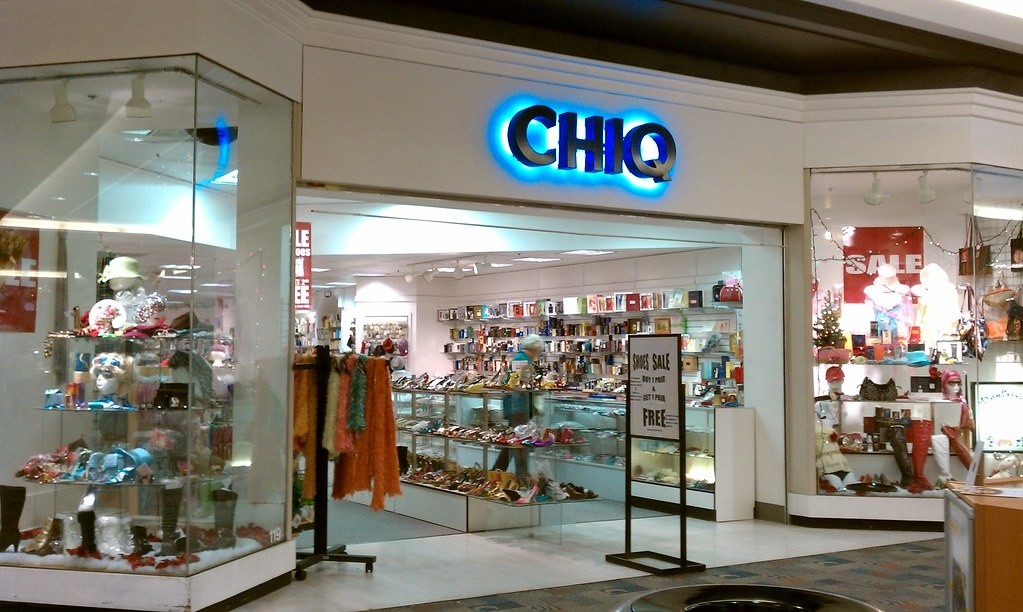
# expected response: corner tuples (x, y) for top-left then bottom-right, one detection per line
(960, 405), (976, 429)
(720, 283), (743, 302)
(957, 215), (1023, 361)
(857, 376), (901, 402)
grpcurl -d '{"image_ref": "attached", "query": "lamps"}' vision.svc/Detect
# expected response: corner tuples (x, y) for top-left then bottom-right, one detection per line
(474, 255), (491, 275)
(125, 79), (152, 117)
(452, 259), (464, 280)
(404, 266), (420, 283)
(864, 171), (883, 205)
(423, 262), (439, 282)
(917, 171), (937, 204)
(961, 172), (982, 207)
(49, 83), (77, 124)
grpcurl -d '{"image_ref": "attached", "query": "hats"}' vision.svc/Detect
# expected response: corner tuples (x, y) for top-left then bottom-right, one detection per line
(96, 257), (145, 284)
(365, 338), (408, 370)
(170, 312), (214, 330)
(826, 366), (845, 382)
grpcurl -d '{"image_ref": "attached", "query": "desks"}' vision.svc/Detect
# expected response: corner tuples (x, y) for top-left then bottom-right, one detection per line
(943, 482), (1023, 612)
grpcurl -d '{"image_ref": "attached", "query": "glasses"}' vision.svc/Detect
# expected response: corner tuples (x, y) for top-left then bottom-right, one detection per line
(949, 381), (962, 386)
(94, 367), (113, 378)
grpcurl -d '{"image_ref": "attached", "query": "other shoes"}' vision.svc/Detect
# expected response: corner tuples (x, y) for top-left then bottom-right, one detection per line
(393, 369), (599, 503)
(859, 473), (892, 487)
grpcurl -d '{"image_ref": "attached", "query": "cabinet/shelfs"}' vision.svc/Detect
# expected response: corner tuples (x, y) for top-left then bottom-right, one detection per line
(815, 356), (970, 495)
(22, 329), (250, 546)
(293, 300), (744, 579)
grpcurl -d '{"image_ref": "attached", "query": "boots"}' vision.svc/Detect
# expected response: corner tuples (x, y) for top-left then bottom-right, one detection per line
(888, 420), (972, 492)
(21, 518), (63, 556)
(153, 485), (184, 557)
(202, 490), (238, 550)
(78, 510), (96, 554)
(131, 525), (153, 555)
(0, 485), (26, 552)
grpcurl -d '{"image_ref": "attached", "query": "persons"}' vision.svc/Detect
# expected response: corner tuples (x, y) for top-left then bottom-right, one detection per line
(864, 265), (912, 338)
(826, 366), (845, 394)
(942, 369), (968, 437)
(88, 352), (132, 400)
(493, 334), (545, 474)
(911, 263), (960, 346)
(97, 257), (147, 291)
(161, 311), (213, 555)
